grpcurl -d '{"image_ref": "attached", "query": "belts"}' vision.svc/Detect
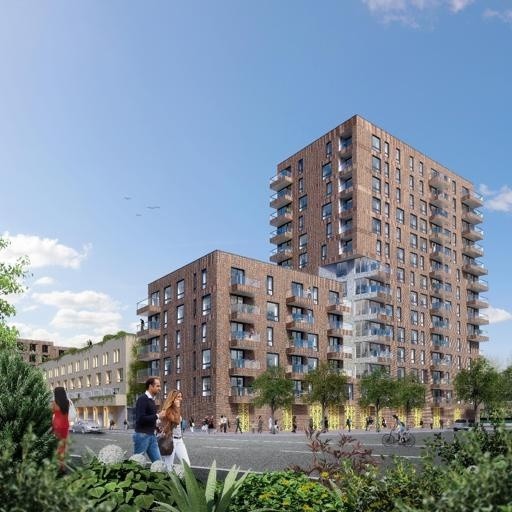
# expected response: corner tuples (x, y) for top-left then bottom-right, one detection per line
(174, 436), (182, 439)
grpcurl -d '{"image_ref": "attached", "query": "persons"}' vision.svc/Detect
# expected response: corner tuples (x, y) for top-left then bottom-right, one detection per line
(51, 385), (72, 475)
(439, 417), (443, 429)
(392, 414), (407, 442)
(132, 375), (167, 463)
(109, 419), (112, 429)
(180, 413), (388, 436)
(419, 417), (424, 428)
(123, 418), (128, 430)
(429, 416), (434, 429)
(111, 419), (115, 429)
(159, 388), (190, 478)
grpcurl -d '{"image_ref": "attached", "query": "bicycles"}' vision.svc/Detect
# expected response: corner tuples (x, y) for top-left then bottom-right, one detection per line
(381, 430), (415, 447)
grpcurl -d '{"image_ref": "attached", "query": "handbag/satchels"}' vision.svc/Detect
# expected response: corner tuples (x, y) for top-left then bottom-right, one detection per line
(158, 436), (173, 456)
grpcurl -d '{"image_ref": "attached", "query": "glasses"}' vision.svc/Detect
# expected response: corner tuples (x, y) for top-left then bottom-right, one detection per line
(173, 390), (179, 394)
